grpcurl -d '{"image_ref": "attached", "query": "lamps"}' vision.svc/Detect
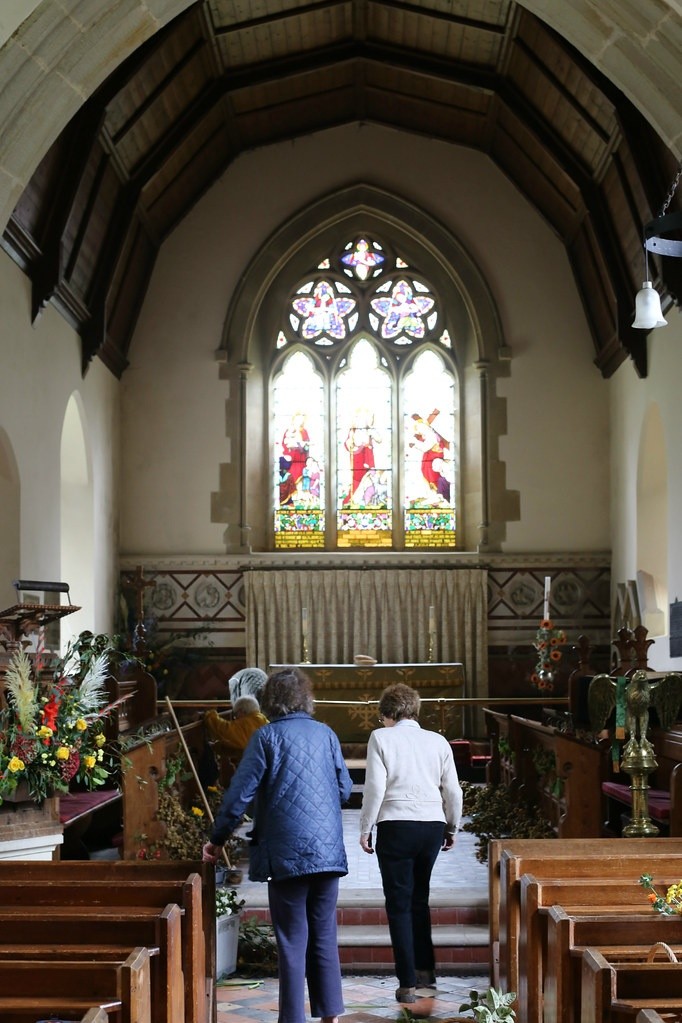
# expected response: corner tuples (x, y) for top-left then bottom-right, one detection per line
(630, 219), (681, 332)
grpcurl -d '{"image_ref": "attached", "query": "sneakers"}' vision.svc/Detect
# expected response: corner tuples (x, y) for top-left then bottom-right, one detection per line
(395, 970), (437, 1003)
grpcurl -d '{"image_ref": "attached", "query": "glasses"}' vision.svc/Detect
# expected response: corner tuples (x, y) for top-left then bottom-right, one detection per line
(232, 710), (240, 719)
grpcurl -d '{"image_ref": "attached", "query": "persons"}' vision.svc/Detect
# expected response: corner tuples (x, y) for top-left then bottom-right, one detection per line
(197, 666), (354, 1023)
(205, 695), (271, 752)
(359, 682), (465, 1003)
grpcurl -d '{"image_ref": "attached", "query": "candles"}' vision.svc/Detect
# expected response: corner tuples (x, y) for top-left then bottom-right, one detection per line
(544, 575), (552, 623)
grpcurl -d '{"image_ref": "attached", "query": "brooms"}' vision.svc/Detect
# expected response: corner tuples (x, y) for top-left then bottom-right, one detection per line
(162, 695), (244, 885)
